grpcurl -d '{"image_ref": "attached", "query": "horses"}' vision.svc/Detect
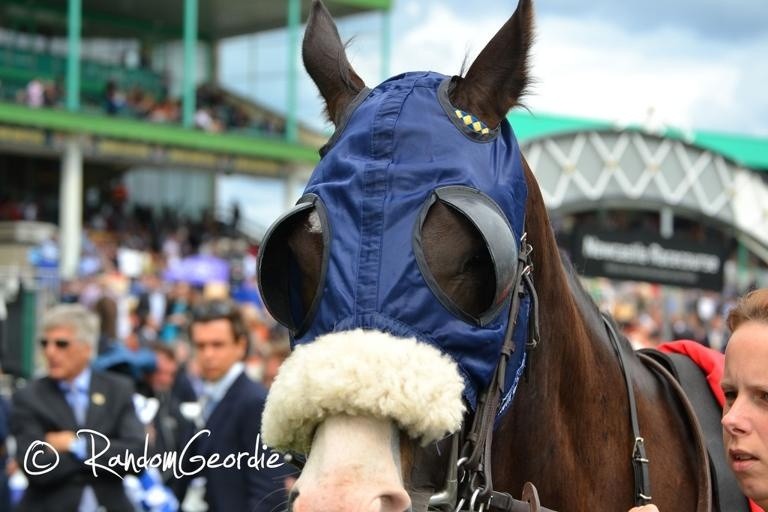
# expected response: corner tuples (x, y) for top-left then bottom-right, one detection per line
(255, 0), (700, 512)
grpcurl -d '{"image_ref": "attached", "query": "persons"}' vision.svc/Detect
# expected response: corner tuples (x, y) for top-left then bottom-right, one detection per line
(625, 287), (768, 512)
(582, 266), (759, 352)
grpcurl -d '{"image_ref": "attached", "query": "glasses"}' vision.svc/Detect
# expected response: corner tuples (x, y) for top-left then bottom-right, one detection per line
(38, 338), (68, 347)
